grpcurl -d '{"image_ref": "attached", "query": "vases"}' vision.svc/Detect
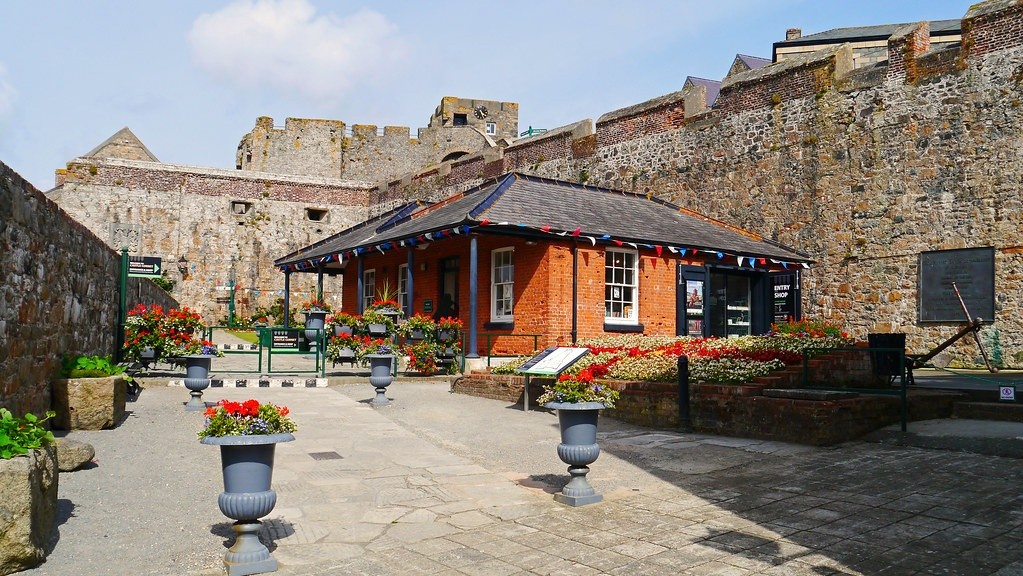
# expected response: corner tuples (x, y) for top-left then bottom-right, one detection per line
(332, 324), (353, 337)
(180, 354), (216, 411)
(406, 329), (424, 340)
(140, 347), (156, 358)
(200, 433), (296, 576)
(255, 326), (266, 337)
(363, 354), (396, 406)
(301, 311), (329, 352)
(366, 324), (386, 336)
(0, 444), (58, 575)
(545, 402), (605, 506)
(435, 329), (455, 341)
(339, 349), (355, 362)
(436, 348), (455, 358)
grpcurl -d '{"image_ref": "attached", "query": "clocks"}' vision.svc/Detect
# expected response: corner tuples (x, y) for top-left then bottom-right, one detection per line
(474, 105), (488, 120)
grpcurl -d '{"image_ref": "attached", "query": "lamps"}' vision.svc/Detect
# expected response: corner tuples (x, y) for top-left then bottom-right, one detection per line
(177, 254), (188, 275)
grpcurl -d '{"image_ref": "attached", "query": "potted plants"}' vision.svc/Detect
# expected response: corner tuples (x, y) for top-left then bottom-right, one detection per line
(52, 355), (133, 431)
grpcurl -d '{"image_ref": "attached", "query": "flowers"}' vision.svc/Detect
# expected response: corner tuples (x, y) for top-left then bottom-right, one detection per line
(0, 408), (56, 459)
(536, 369), (620, 409)
(257, 277), (464, 376)
(122, 304), (225, 369)
(197, 399), (298, 441)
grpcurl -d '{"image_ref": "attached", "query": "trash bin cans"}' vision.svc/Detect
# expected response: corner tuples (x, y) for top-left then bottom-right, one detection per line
(867, 333), (907, 376)
